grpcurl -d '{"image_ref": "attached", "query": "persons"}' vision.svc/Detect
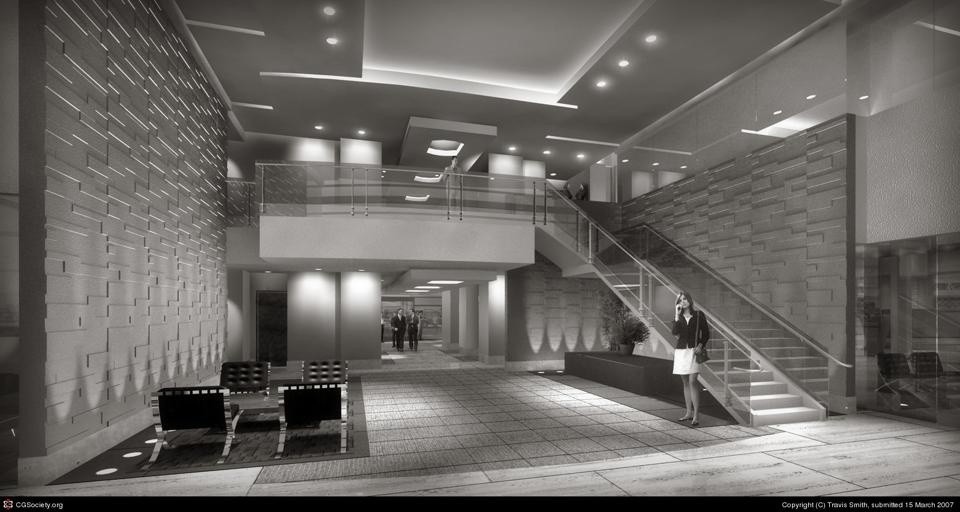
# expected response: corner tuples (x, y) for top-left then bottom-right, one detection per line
(563, 181), (573, 200)
(407, 309), (420, 352)
(668, 290), (710, 426)
(381, 312), (386, 342)
(574, 182), (587, 200)
(393, 308), (407, 353)
(442, 156), (464, 207)
(391, 310), (397, 348)
(417, 309), (426, 340)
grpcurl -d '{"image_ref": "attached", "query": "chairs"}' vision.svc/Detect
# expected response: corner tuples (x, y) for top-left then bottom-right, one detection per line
(145, 359), (354, 463)
(869, 346), (959, 414)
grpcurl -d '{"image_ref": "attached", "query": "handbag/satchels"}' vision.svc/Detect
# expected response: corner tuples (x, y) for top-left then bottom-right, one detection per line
(696, 346), (710, 364)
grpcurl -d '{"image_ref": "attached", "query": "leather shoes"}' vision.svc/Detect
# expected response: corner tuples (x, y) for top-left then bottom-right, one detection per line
(678, 415), (701, 427)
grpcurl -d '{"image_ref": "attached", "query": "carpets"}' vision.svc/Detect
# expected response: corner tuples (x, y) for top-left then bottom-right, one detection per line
(43, 375), (371, 489)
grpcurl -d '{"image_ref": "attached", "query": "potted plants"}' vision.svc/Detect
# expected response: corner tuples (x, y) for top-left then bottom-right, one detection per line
(611, 315), (649, 354)
